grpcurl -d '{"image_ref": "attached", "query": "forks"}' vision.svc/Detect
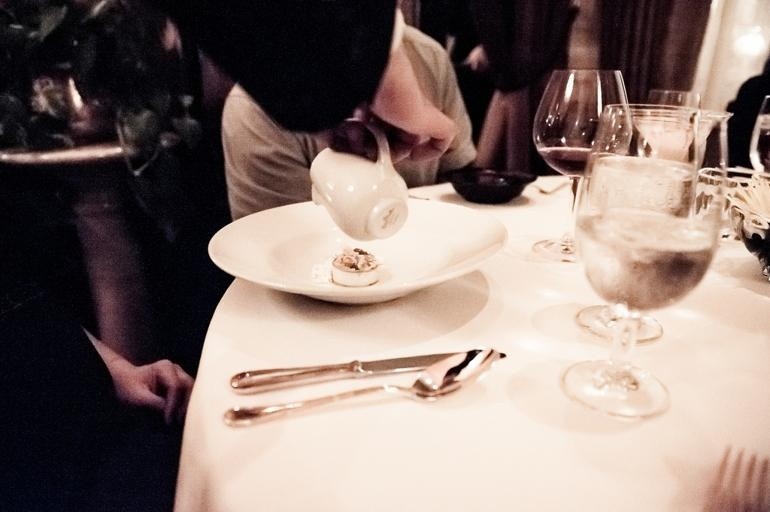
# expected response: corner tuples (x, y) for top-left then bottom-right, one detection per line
(702, 444), (770, 512)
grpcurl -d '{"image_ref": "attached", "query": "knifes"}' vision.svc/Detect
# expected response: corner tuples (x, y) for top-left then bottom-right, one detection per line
(230, 352), (507, 395)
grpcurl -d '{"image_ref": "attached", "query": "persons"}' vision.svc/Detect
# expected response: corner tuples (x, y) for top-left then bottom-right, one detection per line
(1, 1), (396, 510)
(222, 10), (478, 219)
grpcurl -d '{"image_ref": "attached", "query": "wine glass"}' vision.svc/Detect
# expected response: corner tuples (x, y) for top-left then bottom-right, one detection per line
(532, 69), (633, 262)
(562, 90), (732, 420)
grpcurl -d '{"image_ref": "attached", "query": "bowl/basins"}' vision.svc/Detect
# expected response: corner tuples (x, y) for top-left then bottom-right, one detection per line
(444, 167), (537, 204)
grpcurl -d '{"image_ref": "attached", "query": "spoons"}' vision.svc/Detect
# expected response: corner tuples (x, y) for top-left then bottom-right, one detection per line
(224, 348), (500, 427)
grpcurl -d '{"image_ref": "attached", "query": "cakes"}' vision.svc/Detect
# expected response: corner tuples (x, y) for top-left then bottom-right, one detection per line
(331, 247), (379, 288)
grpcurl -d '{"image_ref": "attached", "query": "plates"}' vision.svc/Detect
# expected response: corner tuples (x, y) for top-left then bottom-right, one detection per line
(207, 197), (509, 305)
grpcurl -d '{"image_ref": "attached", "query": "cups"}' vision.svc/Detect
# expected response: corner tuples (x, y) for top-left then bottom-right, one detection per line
(749, 95), (770, 172)
(310, 117), (410, 241)
